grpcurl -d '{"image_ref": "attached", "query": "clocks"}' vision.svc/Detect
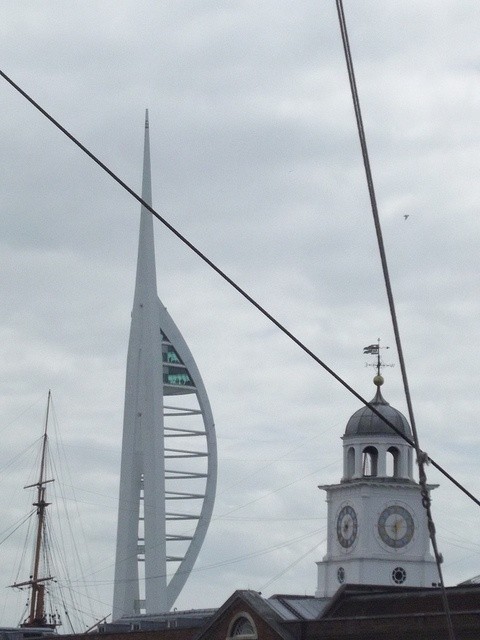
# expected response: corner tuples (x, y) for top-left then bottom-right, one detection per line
(333, 500), (361, 555)
(374, 499), (420, 555)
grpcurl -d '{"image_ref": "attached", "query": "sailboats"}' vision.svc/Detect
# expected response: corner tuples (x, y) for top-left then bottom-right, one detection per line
(0, 389), (96, 640)
